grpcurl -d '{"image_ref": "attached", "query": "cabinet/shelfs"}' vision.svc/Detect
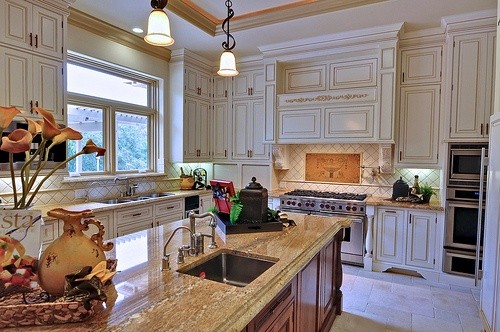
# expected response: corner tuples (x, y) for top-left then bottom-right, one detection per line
(374, 205), (444, 275)
(199, 195), (214, 213)
(0, 0), (74, 177)
(170, 8), (497, 168)
(242, 229), (343, 332)
(42, 199), (185, 258)
(268, 197), (280, 210)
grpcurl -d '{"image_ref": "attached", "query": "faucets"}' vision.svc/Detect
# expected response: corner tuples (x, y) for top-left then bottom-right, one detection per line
(112, 176), (138, 196)
(160, 226), (197, 271)
(189, 210), (218, 257)
(83, 182), (102, 202)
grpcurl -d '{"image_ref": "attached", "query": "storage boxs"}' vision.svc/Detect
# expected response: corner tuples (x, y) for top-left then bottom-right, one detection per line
(210, 178), (236, 214)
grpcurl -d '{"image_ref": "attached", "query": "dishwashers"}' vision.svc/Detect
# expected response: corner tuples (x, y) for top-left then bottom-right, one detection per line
(185, 195), (200, 219)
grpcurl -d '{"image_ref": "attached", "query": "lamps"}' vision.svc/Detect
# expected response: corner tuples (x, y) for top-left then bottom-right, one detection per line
(217, 0), (240, 77)
(143, 0), (175, 47)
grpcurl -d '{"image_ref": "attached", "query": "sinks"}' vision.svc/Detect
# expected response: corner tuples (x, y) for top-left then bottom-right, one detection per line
(99, 199), (130, 204)
(139, 193), (173, 198)
(130, 197), (149, 201)
(175, 248), (280, 287)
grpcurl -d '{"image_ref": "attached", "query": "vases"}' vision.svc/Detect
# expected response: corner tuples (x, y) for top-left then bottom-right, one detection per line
(0, 207), (42, 288)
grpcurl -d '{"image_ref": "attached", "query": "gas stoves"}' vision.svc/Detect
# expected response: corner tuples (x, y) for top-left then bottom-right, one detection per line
(279, 188), (367, 216)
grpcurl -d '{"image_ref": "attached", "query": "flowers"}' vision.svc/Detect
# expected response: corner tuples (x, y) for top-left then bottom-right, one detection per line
(0, 105), (108, 209)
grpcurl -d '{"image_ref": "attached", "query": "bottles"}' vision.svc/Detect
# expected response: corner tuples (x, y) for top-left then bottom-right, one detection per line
(412, 175), (420, 195)
(239, 176), (268, 224)
(38, 207), (114, 296)
(392, 177), (409, 200)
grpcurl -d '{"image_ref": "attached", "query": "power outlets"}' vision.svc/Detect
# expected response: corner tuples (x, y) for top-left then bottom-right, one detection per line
(280, 182), (288, 188)
(74, 189), (85, 199)
(152, 182), (155, 190)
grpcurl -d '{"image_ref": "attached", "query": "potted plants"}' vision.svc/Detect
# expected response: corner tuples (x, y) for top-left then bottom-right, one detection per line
(419, 183), (436, 204)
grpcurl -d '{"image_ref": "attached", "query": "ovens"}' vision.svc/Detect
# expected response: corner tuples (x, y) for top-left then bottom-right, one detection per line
(442, 142), (489, 279)
(282, 211), (366, 266)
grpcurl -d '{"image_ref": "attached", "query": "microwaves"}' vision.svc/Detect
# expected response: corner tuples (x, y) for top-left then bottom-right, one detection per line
(0, 121), (67, 171)
